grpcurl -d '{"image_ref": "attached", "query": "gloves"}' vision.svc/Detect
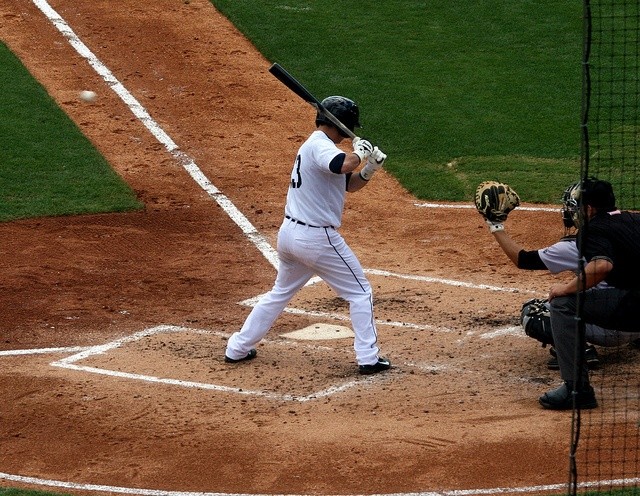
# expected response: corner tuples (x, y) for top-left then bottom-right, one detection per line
(353, 139), (373, 163)
(360, 147), (387, 181)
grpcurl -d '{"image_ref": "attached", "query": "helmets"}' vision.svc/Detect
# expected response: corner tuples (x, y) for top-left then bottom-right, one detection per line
(316, 96), (361, 128)
(561, 175), (597, 228)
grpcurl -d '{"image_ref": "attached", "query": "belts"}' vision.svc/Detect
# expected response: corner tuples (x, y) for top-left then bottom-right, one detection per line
(285, 215), (335, 229)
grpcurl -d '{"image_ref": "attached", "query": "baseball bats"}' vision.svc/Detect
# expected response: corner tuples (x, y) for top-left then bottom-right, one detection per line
(268, 62), (383, 164)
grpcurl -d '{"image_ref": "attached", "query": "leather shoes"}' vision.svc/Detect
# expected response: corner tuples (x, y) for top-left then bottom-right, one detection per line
(224, 349), (257, 363)
(359, 356), (390, 374)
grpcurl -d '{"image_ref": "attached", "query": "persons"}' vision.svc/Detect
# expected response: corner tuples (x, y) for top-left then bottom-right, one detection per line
(224, 96), (391, 374)
(538, 180), (639, 410)
(475, 176), (640, 369)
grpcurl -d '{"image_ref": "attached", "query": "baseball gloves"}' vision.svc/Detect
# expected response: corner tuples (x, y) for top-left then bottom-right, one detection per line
(475, 177), (520, 233)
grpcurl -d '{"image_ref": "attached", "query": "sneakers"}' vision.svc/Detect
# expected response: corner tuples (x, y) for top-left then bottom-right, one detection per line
(539, 382), (598, 410)
(547, 344), (601, 370)
